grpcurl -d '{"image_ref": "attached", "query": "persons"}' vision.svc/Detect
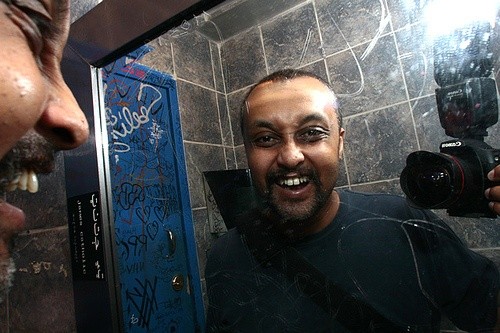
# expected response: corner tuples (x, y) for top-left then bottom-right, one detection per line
(0, 0), (91, 289)
(203, 69), (500, 333)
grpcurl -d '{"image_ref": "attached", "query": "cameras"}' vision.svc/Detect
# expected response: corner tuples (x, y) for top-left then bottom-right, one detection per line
(400, 0), (500, 219)
